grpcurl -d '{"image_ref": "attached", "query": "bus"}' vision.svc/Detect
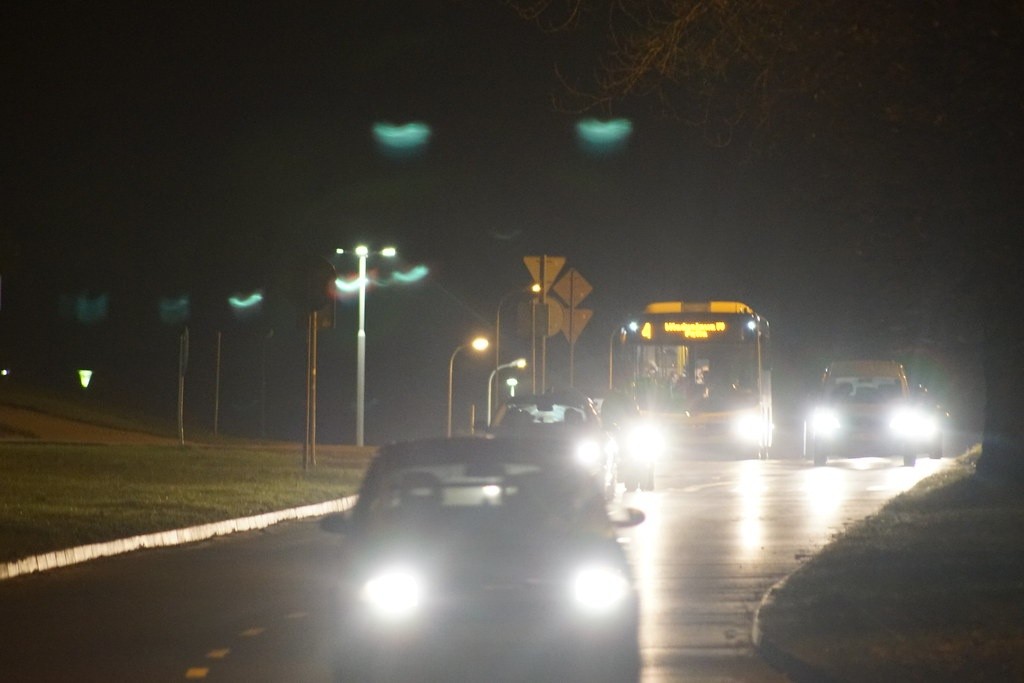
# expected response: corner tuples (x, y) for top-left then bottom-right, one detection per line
(608, 298), (773, 460)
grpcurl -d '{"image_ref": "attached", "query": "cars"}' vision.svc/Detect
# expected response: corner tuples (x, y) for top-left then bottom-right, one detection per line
(482, 393), (613, 506)
(320, 434), (643, 682)
(912, 382), (947, 458)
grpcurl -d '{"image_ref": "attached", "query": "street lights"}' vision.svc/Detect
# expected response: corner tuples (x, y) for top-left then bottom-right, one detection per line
(333, 243), (401, 445)
(445, 337), (491, 438)
(505, 377), (519, 402)
(492, 283), (545, 442)
(488, 358), (529, 434)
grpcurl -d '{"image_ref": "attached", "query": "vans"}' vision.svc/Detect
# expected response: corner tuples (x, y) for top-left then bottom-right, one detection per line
(805, 356), (916, 467)
